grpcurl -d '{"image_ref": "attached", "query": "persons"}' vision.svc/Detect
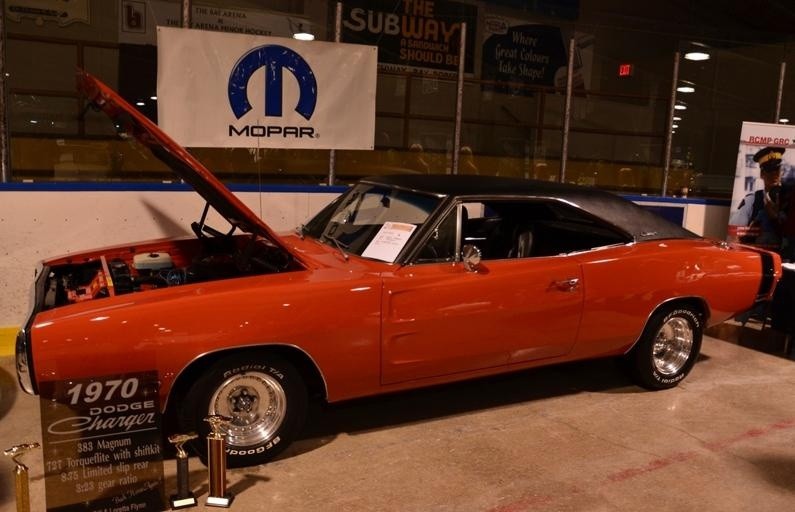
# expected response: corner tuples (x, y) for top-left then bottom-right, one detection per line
(726, 145), (788, 238)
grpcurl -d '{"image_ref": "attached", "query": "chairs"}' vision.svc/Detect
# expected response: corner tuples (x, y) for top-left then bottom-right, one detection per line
(478, 218), (534, 260)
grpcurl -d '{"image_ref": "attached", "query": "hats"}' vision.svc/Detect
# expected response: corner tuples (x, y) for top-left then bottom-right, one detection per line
(752, 146), (786, 167)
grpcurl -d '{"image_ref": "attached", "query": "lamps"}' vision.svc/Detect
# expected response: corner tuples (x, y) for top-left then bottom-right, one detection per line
(676, 82), (695, 93)
(674, 102), (687, 110)
(672, 115), (682, 134)
(684, 44), (710, 61)
(293, 23), (315, 41)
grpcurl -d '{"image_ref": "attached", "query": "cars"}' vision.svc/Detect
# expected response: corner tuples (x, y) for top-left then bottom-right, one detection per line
(15, 66), (783, 470)
